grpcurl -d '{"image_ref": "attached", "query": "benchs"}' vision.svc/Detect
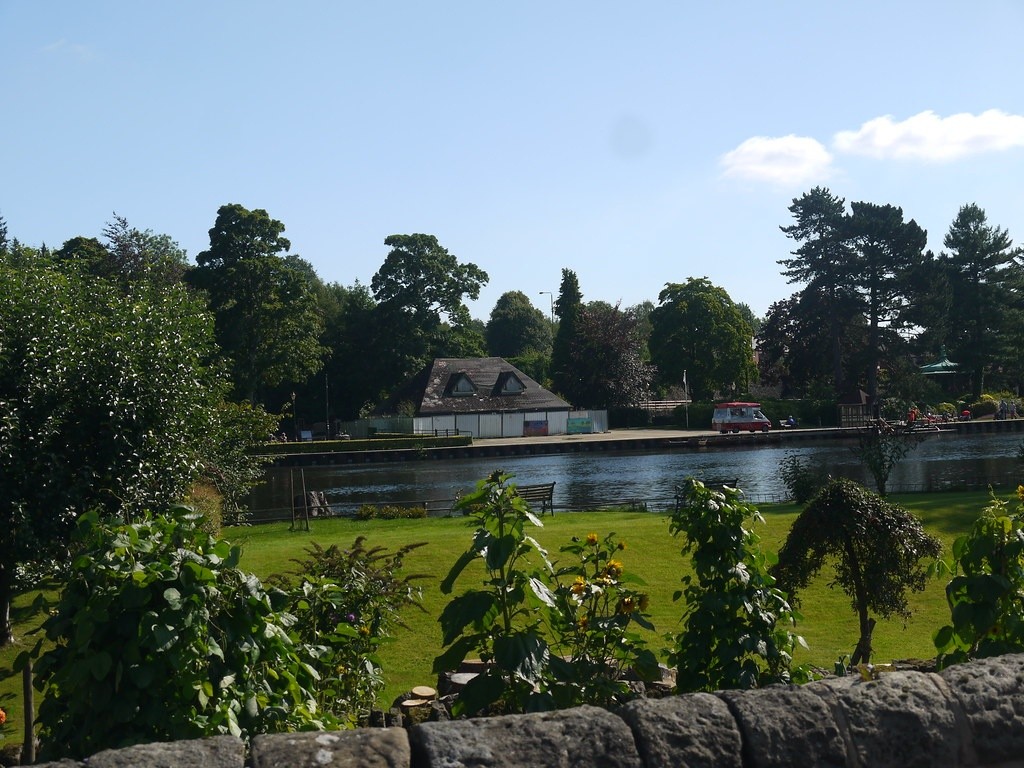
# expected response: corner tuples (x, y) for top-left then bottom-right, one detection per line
(779, 420), (792, 430)
(504, 481), (556, 518)
(673, 477), (739, 513)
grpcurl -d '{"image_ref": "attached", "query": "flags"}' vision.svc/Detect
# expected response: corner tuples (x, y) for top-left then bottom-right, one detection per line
(682, 372), (686, 382)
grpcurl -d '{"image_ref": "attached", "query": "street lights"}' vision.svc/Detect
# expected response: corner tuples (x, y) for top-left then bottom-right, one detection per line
(539, 292), (553, 332)
(290, 392), (299, 442)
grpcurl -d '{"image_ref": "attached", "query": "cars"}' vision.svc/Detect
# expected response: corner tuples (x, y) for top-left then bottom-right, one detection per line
(712, 403), (771, 434)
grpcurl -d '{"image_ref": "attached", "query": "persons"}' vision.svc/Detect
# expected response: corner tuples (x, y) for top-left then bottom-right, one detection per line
(927, 410), (935, 423)
(787, 415), (797, 429)
(999, 399), (1006, 419)
(943, 410), (948, 422)
(269, 434), (276, 442)
(962, 409), (970, 416)
(911, 406), (917, 427)
(755, 413), (762, 419)
(280, 433), (287, 442)
(1010, 402), (1015, 419)
(731, 409), (736, 414)
(993, 412), (999, 420)
